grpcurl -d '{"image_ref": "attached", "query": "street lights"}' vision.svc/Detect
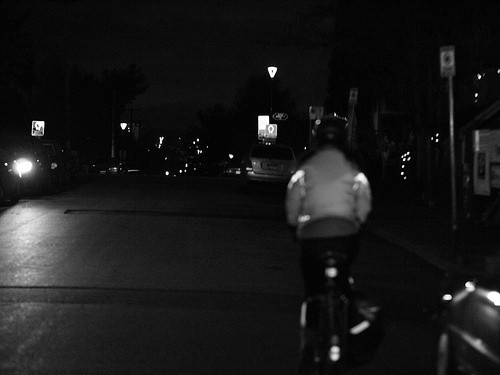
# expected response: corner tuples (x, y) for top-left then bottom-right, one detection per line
(437, 43), (460, 235)
(268, 66), (278, 123)
(120, 122), (127, 168)
(230, 154), (234, 159)
(159, 136), (164, 170)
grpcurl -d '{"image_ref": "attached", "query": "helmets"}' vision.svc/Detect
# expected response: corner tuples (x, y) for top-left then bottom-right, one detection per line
(318, 119), (345, 143)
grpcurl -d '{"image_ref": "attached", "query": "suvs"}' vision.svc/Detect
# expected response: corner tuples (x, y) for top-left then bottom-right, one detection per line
(109, 166), (119, 175)
(223, 162), (241, 176)
(238, 140), (297, 186)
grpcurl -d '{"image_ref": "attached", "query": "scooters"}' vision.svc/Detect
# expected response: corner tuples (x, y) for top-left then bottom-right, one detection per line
(299, 246), (361, 375)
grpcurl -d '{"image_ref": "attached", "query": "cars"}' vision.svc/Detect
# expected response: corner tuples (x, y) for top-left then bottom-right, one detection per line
(92, 165), (105, 175)
(0, 137), (65, 202)
(434, 284), (499, 375)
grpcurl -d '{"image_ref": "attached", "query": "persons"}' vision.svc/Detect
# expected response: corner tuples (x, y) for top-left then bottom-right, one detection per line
(283, 146), (371, 327)
